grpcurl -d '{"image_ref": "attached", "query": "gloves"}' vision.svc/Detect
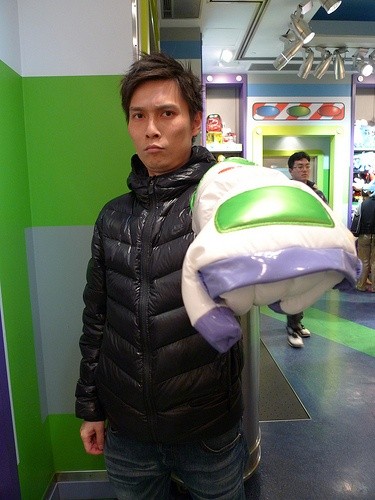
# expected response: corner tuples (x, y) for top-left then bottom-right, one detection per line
(179, 153), (362, 357)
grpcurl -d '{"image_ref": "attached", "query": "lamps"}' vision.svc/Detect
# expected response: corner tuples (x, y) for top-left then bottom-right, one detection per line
(272, 0), (375, 80)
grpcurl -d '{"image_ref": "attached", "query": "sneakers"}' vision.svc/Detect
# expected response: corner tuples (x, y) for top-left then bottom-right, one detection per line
(286, 332), (303, 349)
(300, 326), (313, 338)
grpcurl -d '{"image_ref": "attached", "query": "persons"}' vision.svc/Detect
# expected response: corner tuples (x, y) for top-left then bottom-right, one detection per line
(286, 152), (329, 348)
(354, 195), (375, 292)
(72, 50), (251, 500)
